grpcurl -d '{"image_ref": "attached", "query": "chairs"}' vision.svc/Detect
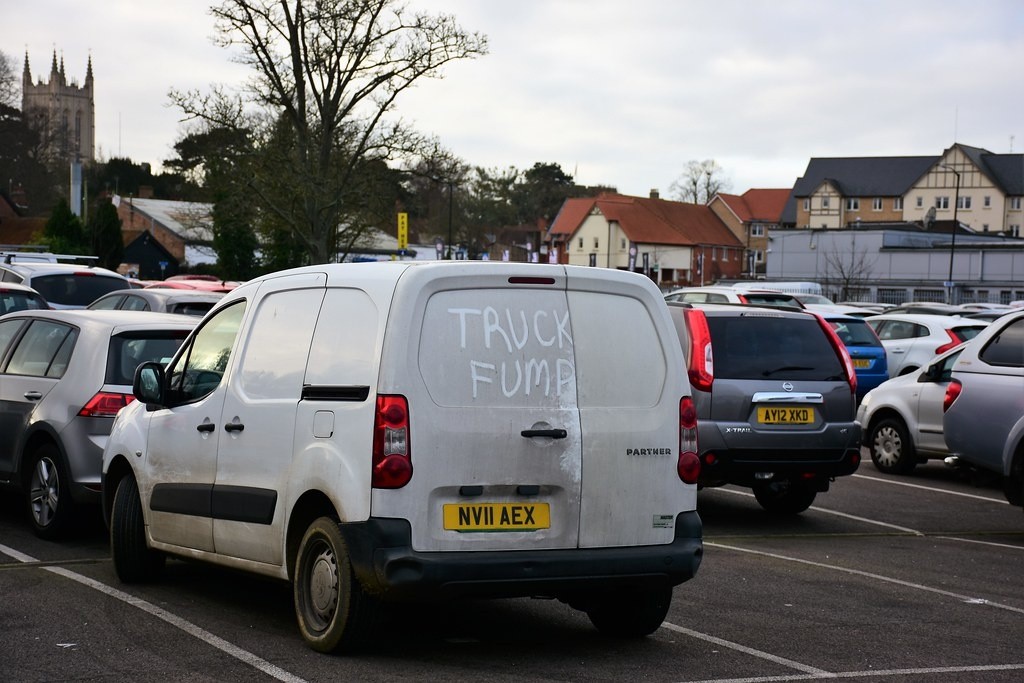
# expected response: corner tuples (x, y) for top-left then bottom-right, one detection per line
(46, 279), (70, 302)
(891, 325), (913, 338)
(43, 335), (73, 378)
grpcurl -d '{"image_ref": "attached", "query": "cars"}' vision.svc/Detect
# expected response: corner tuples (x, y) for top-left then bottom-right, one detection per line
(1, 310), (206, 540)
(0, 283), (50, 315)
(855, 337), (973, 475)
(53, 272), (245, 374)
(0, 253), (133, 311)
(660, 277), (1024, 410)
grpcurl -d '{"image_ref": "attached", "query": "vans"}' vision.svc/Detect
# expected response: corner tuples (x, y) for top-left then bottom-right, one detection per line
(100, 259), (709, 656)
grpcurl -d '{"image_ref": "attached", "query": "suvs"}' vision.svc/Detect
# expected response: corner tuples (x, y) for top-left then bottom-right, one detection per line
(941, 310), (1024, 508)
(666, 299), (864, 520)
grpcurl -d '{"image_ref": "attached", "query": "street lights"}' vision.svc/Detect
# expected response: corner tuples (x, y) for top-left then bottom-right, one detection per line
(940, 164), (961, 302)
